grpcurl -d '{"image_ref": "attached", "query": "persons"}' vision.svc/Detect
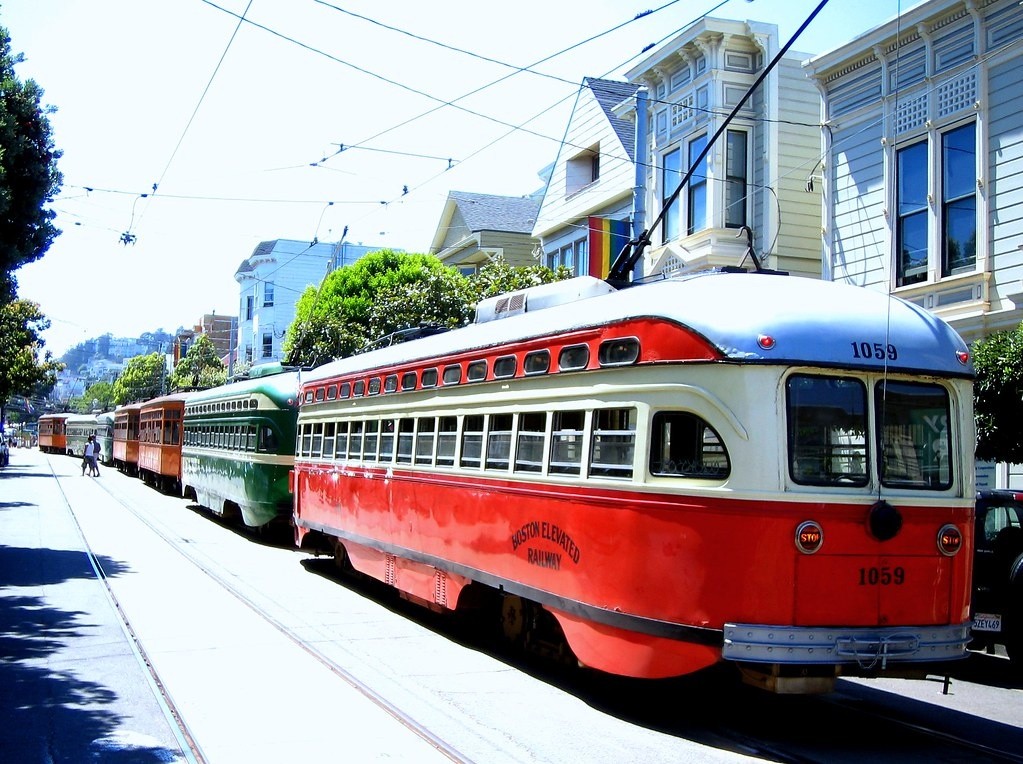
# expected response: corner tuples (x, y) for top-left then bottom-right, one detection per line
(9, 437), (12, 447)
(388, 422), (394, 431)
(81, 435), (101, 477)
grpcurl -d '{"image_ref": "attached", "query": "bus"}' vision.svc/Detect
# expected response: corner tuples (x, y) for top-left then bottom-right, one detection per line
(180, 361), (303, 541)
(138, 392), (189, 496)
(285, 225), (979, 745)
(95, 412), (117, 467)
(65, 412), (96, 458)
(37, 413), (72, 454)
(112, 403), (143, 477)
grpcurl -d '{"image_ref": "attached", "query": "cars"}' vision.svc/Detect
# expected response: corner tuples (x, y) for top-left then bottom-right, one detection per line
(0, 421), (39, 467)
(969, 488), (1023, 664)
